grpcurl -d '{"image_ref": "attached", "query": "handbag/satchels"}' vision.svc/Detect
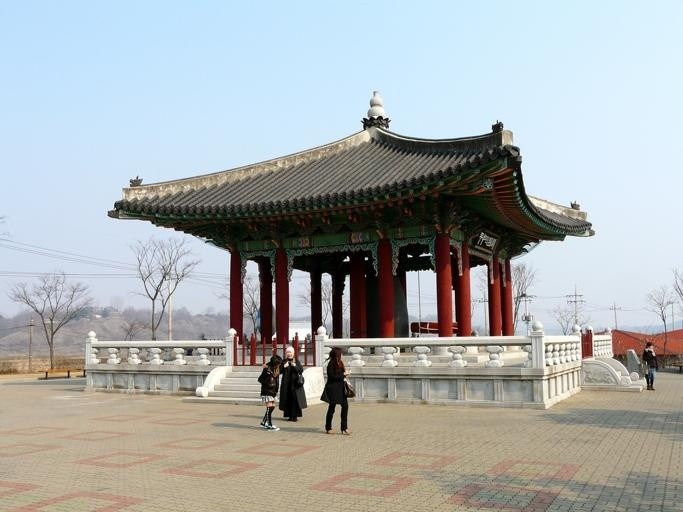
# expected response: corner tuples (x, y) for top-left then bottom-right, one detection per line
(344, 377), (355, 397)
(642, 362), (649, 374)
(295, 373), (304, 387)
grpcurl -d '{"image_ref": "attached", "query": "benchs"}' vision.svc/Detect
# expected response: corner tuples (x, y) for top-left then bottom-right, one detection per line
(672, 362), (683, 372)
(38, 368), (85, 379)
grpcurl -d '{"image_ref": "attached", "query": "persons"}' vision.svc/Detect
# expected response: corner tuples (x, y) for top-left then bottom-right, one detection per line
(319, 347), (355, 434)
(257, 354), (282, 431)
(278, 346), (307, 421)
(640, 342), (656, 390)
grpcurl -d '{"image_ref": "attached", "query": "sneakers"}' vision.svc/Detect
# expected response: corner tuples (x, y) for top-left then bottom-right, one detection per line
(288, 417), (297, 421)
(261, 422), (276, 429)
(647, 385), (654, 390)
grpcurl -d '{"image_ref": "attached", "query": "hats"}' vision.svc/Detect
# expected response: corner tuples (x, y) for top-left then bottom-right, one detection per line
(286, 347), (294, 353)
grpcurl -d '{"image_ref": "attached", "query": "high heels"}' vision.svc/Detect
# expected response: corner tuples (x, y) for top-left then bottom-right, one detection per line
(327, 429), (349, 434)
